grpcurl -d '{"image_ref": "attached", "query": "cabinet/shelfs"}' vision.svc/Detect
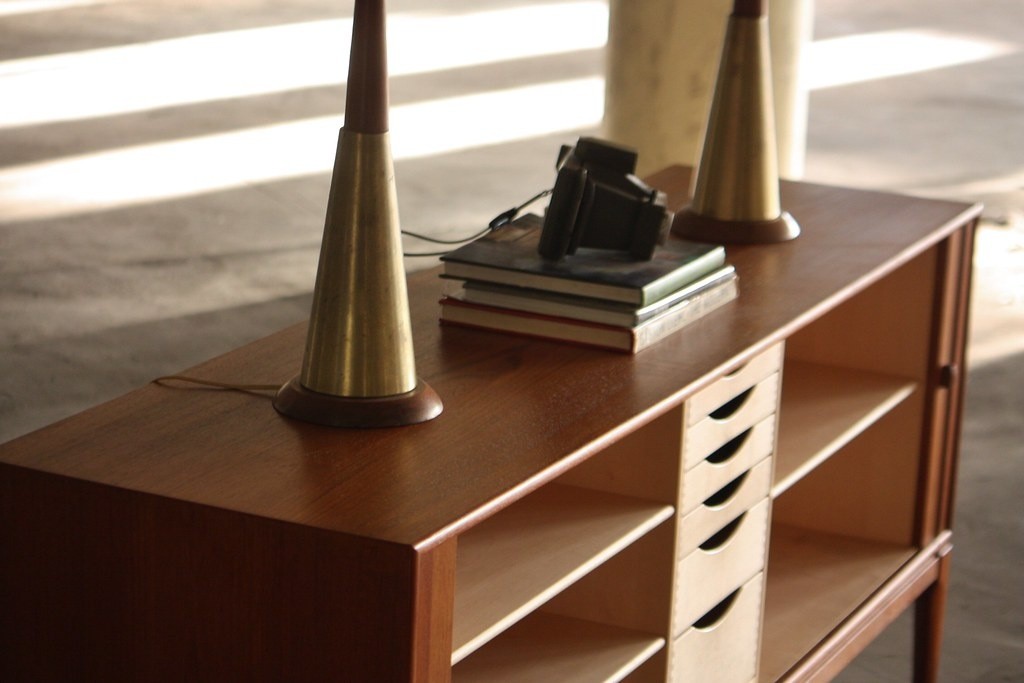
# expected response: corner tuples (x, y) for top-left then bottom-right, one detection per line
(0, 160), (989, 683)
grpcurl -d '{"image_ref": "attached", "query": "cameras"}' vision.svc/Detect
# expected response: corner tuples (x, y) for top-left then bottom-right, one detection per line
(537, 135), (667, 262)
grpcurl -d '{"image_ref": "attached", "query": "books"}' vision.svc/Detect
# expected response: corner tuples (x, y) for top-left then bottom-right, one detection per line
(437, 209), (739, 354)
